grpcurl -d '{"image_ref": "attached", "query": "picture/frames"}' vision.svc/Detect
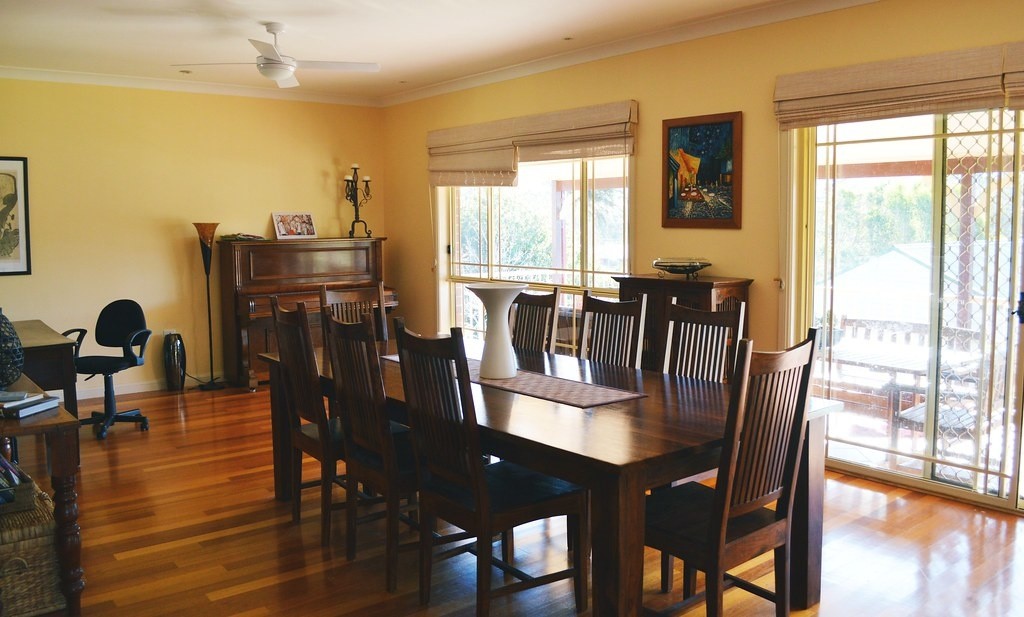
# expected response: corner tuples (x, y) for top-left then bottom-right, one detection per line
(661, 111), (742, 230)
(0, 156), (32, 276)
(272, 212), (317, 240)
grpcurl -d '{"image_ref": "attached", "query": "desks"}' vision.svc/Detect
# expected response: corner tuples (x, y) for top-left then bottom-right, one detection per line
(611, 274), (754, 383)
(0, 373), (85, 617)
(257, 339), (844, 617)
(818, 336), (975, 468)
(0, 319), (81, 468)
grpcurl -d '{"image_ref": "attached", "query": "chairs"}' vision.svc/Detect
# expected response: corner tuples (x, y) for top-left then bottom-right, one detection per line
(576, 289), (647, 369)
(508, 287), (561, 353)
(894, 351), (995, 465)
(642, 327), (820, 617)
(319, 281), (389, 419)
(270, 294), (419, 547)
(656, 295), (746, 594)
(61, 299), (152, 441)
(393, 316), (591, 617)
(321, 304), (489, 594)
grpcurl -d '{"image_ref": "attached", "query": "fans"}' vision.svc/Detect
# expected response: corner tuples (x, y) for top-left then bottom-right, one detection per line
(170, 21), (381, 88)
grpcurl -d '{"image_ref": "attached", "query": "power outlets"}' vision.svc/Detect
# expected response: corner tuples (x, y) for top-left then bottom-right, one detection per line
(163, 329), (176, 338)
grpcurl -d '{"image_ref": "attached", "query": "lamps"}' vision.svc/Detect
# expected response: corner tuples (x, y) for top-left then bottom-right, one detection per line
(192, 222), (225, 391)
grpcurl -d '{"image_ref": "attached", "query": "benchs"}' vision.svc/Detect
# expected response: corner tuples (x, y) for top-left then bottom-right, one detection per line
(825, 315), (980, 400)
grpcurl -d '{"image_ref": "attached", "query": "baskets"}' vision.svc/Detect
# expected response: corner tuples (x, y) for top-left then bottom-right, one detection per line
(0, 461), (69, 617)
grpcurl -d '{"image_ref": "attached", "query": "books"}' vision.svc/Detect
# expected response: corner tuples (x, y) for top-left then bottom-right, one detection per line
(0, 390), (43, 408)
(0, 454), (23, 505)
(0, 396), (60, 419)
